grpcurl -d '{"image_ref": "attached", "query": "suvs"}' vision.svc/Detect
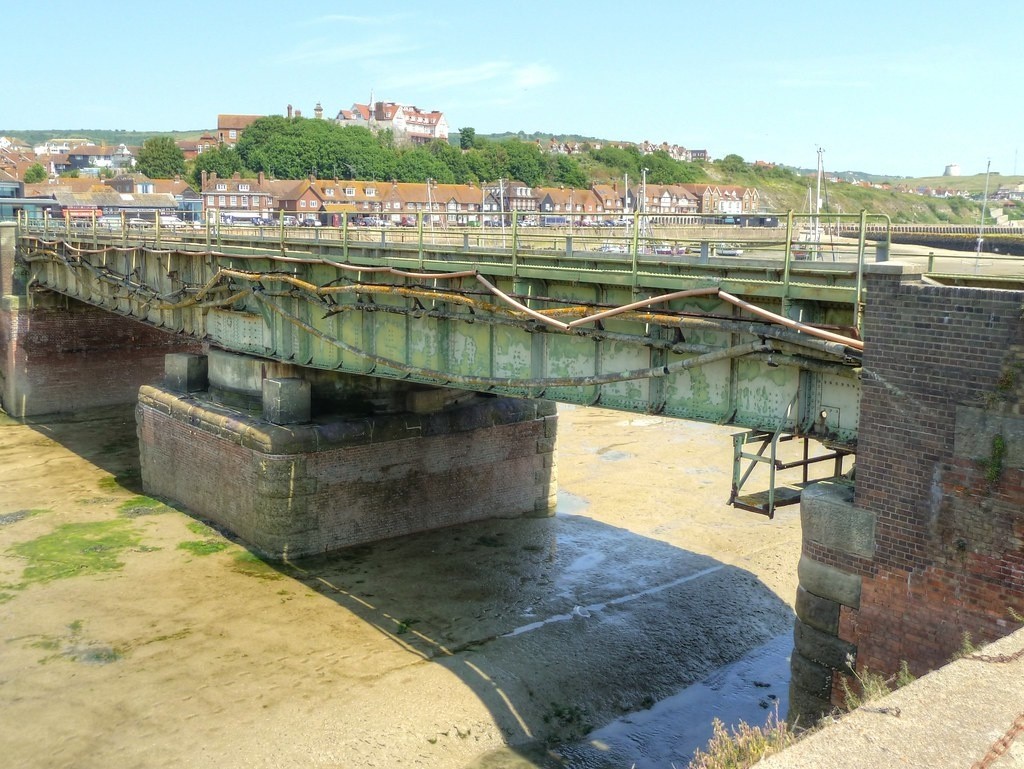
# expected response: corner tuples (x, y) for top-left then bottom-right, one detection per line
(127, 218), (154, 229)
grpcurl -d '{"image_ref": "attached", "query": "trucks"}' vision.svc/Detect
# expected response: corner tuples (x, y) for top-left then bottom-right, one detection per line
(541, 215), (571, 228)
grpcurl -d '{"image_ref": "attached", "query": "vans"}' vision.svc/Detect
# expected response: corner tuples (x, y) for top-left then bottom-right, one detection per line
(282, 215), (301, 226)
(160, 216), (187, 228)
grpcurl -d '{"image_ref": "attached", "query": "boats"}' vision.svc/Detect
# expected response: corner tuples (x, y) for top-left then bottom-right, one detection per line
(600, 167), (711, 254)
(715, 248), (743, 257)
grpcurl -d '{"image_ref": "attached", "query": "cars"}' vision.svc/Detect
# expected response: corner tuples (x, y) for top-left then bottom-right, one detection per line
(346, 216), (427, 227)
(466, 219), (538, 227)
(303, 218), (322, 227)
(573, 221), (589, 227)
(616, 219), (631, 226)
(591, 221), (604, 227)
(604, 220), (615, 227)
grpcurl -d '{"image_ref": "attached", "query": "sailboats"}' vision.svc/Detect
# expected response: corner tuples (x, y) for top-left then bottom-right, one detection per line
(792, 187), (823, 260)
